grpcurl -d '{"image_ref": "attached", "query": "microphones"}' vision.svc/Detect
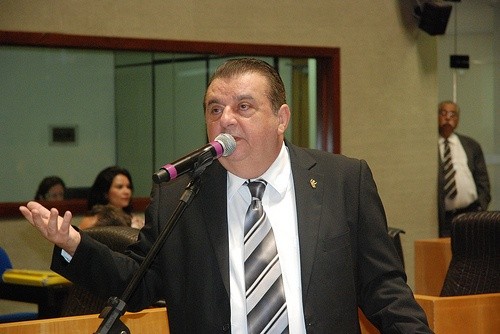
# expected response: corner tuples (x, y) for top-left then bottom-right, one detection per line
(152, 133), (236, 184)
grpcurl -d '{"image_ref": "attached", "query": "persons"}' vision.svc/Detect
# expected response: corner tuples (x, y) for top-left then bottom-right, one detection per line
(35, 176), (69, 202)
(19, 59), (433, 334)
(437, 101), (491, 238)
(78, 166), (144, 228)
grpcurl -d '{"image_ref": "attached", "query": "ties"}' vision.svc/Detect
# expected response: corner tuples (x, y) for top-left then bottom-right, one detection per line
(442, 140), (457, 200)
(243, 178), (289, 334)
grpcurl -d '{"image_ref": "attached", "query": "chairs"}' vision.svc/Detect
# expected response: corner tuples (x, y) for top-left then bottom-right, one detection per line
(60, 226), (141, 317)
(439, 210), (500, 297)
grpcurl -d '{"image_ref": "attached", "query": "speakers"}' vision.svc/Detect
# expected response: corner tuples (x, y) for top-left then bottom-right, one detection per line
(418, 0), (452, 36)
